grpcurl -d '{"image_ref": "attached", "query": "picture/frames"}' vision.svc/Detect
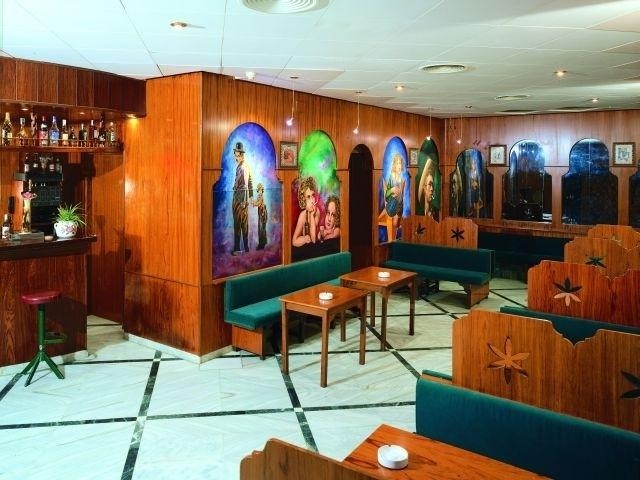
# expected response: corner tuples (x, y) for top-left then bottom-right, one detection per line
(278, 141), (299, 170)
(489, 145), (507, 167)
(612, 142), (636, 167)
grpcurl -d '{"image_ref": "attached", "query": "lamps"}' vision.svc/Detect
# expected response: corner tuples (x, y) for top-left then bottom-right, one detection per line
(287, 77), (462, 144)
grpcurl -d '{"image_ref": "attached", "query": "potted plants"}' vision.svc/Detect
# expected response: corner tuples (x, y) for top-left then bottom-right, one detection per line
(49, 201), (89, 238)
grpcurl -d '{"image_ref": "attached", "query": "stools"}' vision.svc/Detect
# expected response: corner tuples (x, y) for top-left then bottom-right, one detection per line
(21, 290), (68, 387)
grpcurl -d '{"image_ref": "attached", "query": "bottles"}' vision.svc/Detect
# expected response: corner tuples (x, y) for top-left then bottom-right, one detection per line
(2, 213), (11, 242)
(23, 152), (62, 177)
(31, 182), (49, 201)
(1, 112), (119, 146)
